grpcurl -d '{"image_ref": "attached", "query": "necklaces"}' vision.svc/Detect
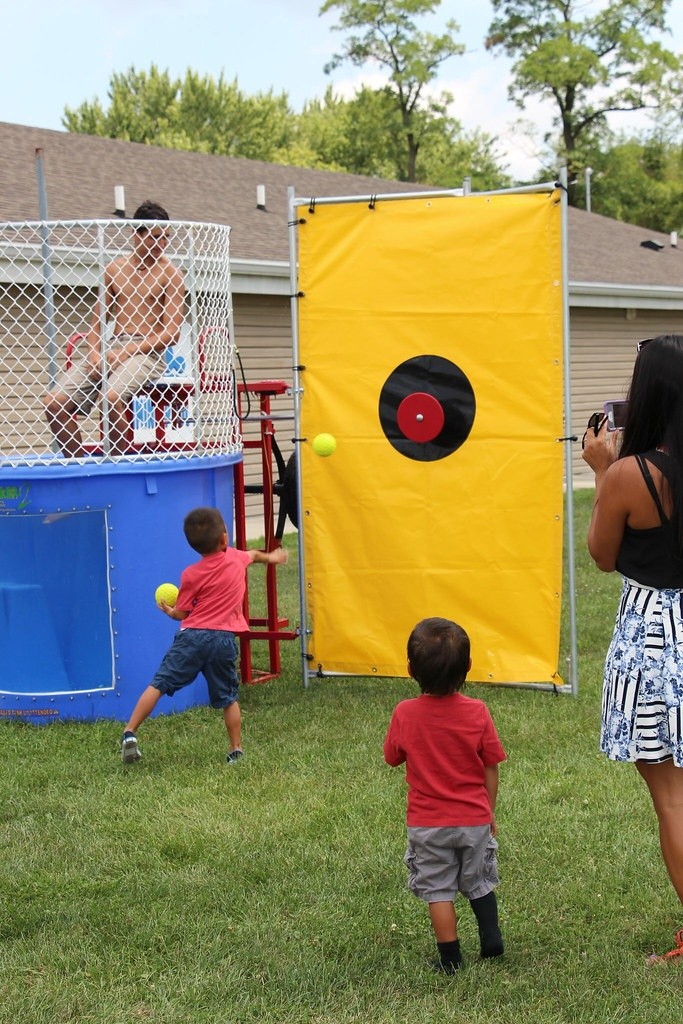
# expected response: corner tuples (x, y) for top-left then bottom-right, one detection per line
(137, 267), (146, 274)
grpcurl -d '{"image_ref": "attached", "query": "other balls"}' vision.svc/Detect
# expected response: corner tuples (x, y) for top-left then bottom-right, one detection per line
(155, 580), (179, 610)
(311, 433), (338, 457)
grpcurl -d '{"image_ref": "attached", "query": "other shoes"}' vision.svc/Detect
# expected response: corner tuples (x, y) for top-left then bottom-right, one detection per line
(121, 731), (142, 763)
(648, 930), (683, 963)
(227, 750), (243, 763)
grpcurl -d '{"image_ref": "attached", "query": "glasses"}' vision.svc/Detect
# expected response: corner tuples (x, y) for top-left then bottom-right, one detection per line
(582, 413), (608, 449)
(637, 338), (655, 354)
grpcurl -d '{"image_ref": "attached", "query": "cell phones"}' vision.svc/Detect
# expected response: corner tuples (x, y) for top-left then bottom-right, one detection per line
(604, 400), (627, 432)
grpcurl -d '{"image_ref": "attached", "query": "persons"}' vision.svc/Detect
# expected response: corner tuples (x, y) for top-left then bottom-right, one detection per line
(384, 618), (506, 975)
(582, 336), (683, 964)
(121, 509), (288, 765)
(43, 199), (186, 457)
